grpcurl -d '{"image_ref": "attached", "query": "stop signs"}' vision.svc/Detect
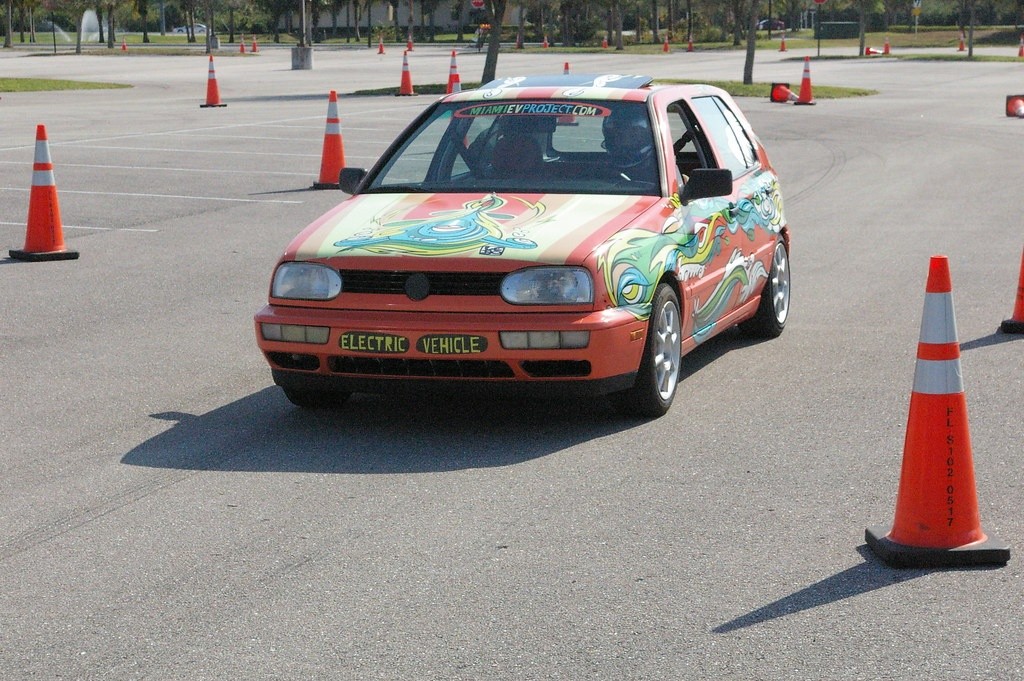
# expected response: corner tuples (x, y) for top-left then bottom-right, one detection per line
(471, 0), (485, 8)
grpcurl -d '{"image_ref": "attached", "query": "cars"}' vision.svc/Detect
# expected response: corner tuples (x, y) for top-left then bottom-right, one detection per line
(755, 20), (785, 31)
(173, 23), (212, 35)
(254, 74), (790, 419)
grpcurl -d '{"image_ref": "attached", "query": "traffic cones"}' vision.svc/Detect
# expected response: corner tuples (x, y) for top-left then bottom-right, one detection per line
(556, 63), (579, 126)
(957, 35), (967, 51)
(1018, 33), (1024, 57)
(882, 39), (889, 55)
(1000, 246), (1024, 334)
(240, 35), (245, 53)
(865, 255), (1011, 570)
(200, 54), (227, 107)
(122, 38), (127, 51)
(309, 91), (345, 190)
(779, 34), (786, 52)
(663, 36), (669, 52)
(687, 38), (693, 52)
(407, 36), (414, 52)
(251, 34), (258, 52)
(544, 35), (548, 48)
(446, 50), (460, 94)
(396, 50), (418, 96)
(865, 47), (879, 56)
(378, 36), (384, 55)
(770, 83), (800, 102)
(603, 37), (608, 49)
(794, 55), (816, 105)
(1006, 95), (1024, 118)
(9, 125), (80, 262)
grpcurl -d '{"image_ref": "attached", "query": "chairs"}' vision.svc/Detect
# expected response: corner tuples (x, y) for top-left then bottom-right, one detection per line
(493, 137), (543, 177)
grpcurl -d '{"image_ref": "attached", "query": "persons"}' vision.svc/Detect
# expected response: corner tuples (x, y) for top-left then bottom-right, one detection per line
(602, 109), (656, 182)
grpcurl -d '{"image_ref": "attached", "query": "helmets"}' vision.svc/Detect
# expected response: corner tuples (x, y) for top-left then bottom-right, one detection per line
(602, 106), (654, 171)
